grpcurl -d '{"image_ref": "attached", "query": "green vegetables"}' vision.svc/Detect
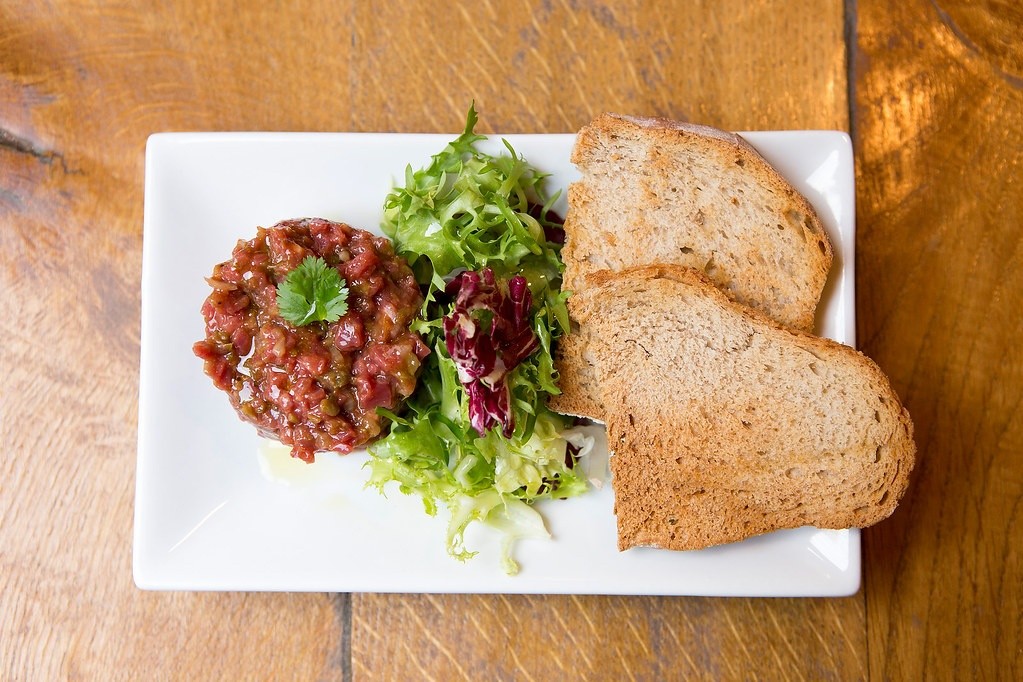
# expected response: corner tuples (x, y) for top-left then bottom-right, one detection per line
(273, 98), (604, 575)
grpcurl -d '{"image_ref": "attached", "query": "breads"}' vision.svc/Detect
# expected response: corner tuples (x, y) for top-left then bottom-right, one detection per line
(587, 263), (916, 552)
(543, 114), (834, 425)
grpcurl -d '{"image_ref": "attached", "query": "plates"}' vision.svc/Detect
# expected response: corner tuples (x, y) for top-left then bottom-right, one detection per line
(131, 132), (862, 596)
